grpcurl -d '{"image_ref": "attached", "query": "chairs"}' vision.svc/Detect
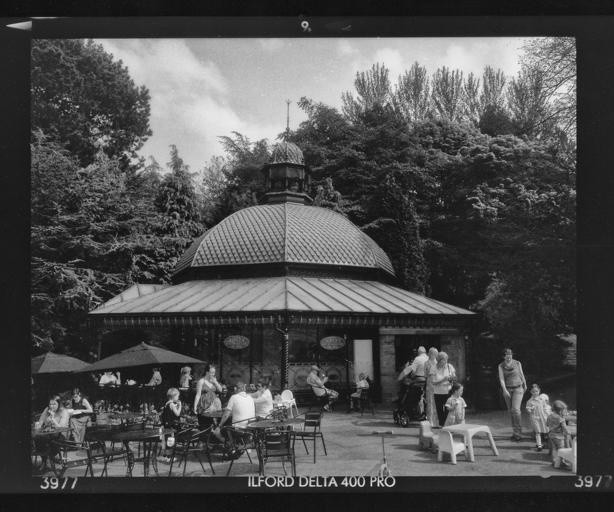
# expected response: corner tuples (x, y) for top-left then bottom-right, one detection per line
(421, 417), (464, 464)
(36, 393), (327, 479)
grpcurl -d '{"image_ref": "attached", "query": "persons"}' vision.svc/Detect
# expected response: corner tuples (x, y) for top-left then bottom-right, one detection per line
(307, 364), (341, 413)
(539, 392), (553, 425)
(65, 386), (94, 452)
(497, 348), (527, 443)
(547, 400), (576, 469)
(525, 384), (553, 451)
(405, 345), (468, 428)
(33, 394), (71, 473)
(348, 373), (370, 413)
(98, 363), (274, 463)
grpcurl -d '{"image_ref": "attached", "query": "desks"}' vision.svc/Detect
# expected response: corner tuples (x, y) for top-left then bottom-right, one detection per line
(447, 421), (500, 462)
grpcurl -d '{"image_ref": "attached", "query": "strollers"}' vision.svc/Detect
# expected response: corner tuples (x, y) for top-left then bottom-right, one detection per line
(390, 378), (431, 427)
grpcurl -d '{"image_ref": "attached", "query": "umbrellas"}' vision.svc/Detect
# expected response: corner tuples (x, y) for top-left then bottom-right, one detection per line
(79, 340), (206, 372)
(31, 351), (92, 374)
